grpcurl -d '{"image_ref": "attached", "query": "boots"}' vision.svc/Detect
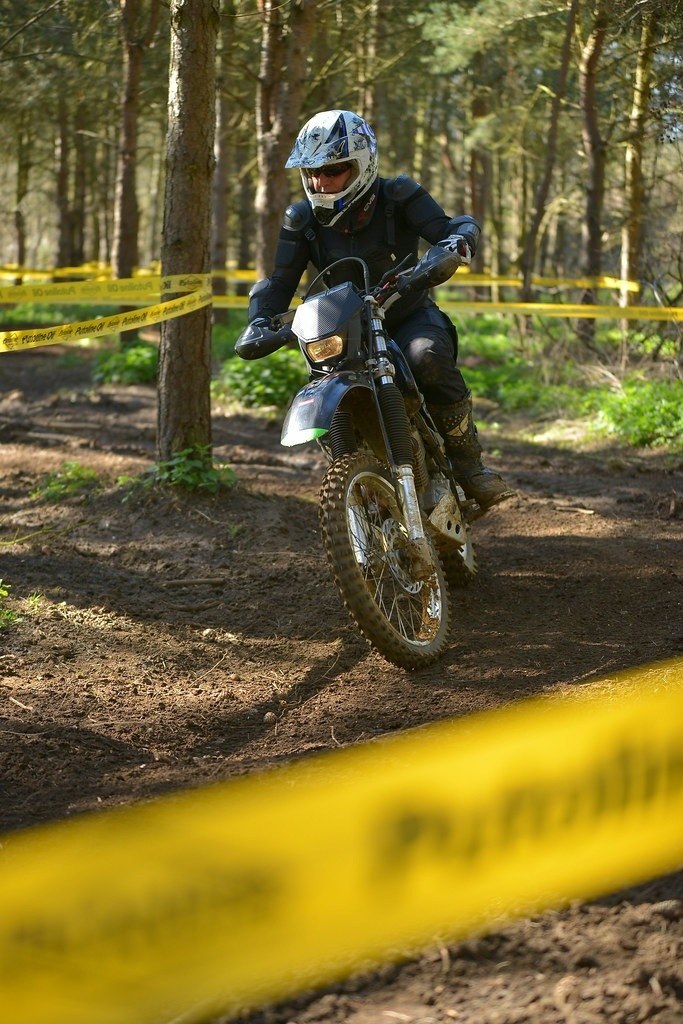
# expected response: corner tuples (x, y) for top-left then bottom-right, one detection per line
(425, 389), (515, 510)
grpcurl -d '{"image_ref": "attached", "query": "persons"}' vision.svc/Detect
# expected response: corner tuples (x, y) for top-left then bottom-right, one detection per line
(238, 111), (514, 507)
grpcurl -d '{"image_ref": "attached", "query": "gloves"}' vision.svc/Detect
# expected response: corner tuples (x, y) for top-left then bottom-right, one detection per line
(438, 235), (470, 267)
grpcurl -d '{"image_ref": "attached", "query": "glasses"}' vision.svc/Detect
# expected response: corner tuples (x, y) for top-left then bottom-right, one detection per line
(306, 162), (352, 179)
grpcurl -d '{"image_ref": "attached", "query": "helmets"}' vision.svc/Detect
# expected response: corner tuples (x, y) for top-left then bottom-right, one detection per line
(284, 109), (378, 228)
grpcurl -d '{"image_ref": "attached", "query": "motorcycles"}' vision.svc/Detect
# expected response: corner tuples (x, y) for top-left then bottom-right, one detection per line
(232, 241), (487, 675)
